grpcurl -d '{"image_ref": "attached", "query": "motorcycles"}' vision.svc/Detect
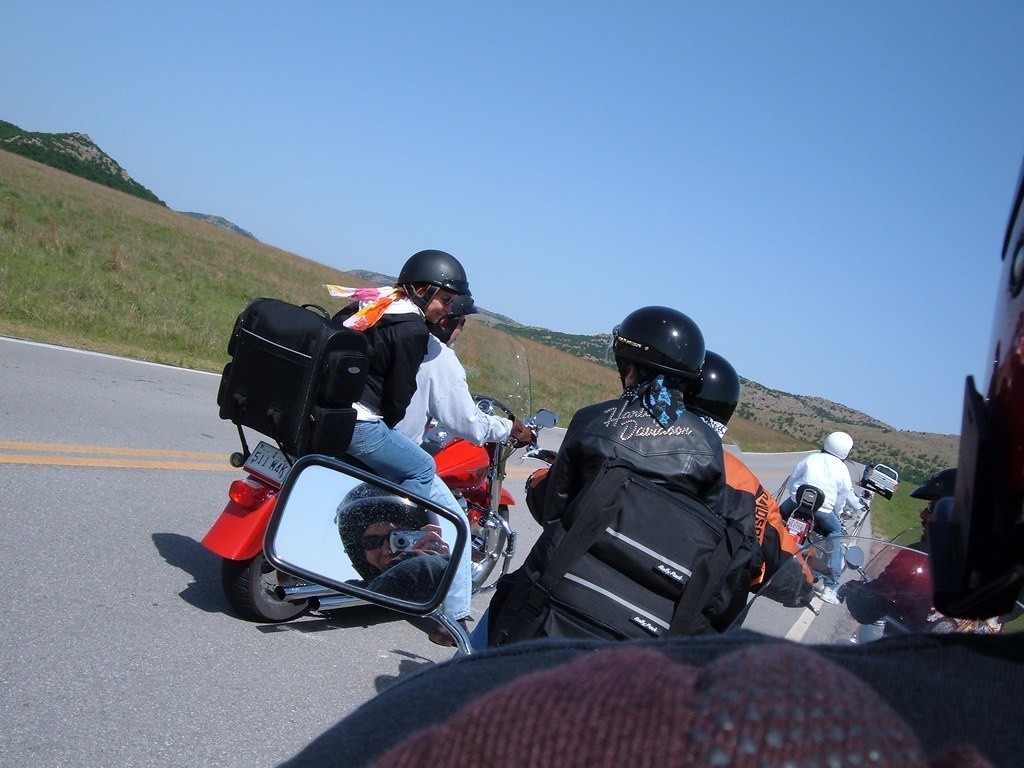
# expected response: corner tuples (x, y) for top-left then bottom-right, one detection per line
(762, 474), (875, 610)
(452, 441), (820, 663)
(834, 526), (1007, 648)
(199, 326), (560, 626)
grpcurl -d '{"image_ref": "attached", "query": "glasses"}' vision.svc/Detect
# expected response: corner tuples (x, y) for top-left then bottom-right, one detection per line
(435, 292), (455, 306)
(459, 318), (466, 327)
(358, 529), (392, 550)
(928, 500), (936, 513)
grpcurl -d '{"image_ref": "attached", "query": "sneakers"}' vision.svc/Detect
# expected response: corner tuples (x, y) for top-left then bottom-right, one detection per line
(821, 587), (839, 605)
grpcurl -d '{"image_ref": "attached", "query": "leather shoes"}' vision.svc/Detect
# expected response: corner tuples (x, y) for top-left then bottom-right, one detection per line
(428, 615), (475, 647)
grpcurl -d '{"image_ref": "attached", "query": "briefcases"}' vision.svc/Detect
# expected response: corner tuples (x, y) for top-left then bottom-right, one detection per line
(216, 297), (369, 457)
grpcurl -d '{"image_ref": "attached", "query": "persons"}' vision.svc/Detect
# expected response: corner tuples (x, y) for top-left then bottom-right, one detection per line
(394, 289), (537, 647)
(323, 249), (436, 501)
(280, 163), (1024, 768)
(779, 432), (869, 605)
(836, 467), (1004, 637)
(333, 481), (453, 604)
(455, 304), (815, 656)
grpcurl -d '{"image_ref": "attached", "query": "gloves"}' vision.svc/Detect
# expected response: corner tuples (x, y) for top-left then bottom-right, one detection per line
(525, 467), (549, 492)
(783, 587), (814, 608)
(507, 413), (538, 448)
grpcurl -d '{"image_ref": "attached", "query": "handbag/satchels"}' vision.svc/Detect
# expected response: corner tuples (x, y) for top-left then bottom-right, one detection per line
(542, 456), (751, 644)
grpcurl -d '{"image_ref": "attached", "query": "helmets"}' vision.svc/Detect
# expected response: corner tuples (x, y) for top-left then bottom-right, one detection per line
(684, 350), (740, 426)
(824, 431), (855, 460)
(931, 160), (1024, 623)
(614, 306), (706, 380)
(398, 249), (471, 294)
(423, 295), (474, 344)
(908, 467), (957, 547)
(336, 483), (440, 579)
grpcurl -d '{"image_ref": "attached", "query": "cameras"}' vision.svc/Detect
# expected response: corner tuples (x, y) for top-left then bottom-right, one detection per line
(389, 530), (439, 555)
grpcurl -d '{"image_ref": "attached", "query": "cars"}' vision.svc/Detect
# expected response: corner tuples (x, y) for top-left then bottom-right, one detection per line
(860, 464), (899, 501)
